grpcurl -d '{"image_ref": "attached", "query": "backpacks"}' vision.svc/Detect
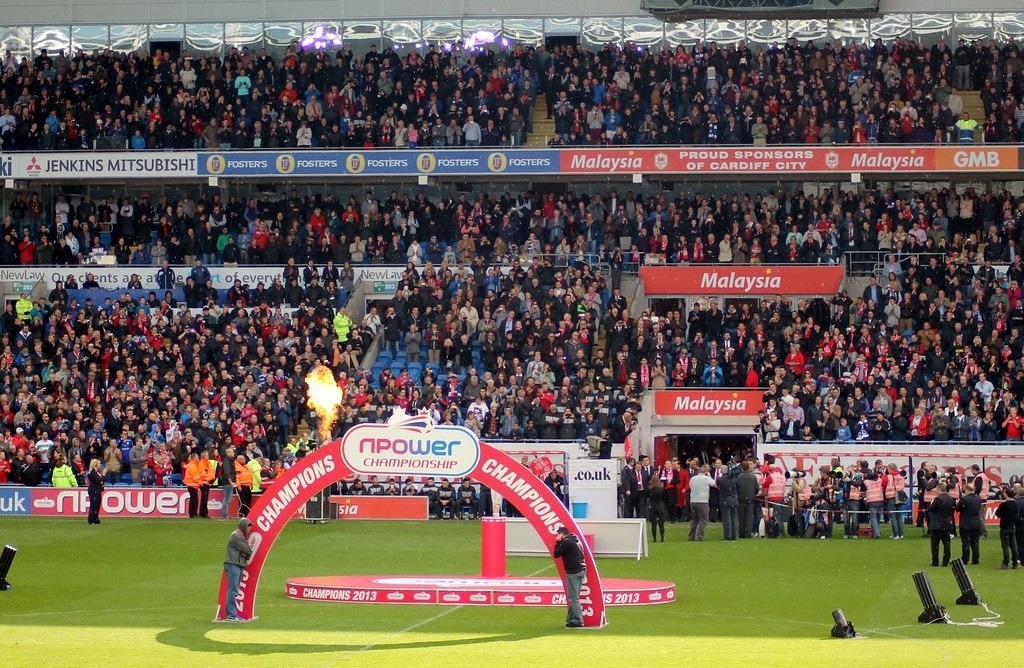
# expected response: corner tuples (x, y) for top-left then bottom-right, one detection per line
(765, 518), (780, 538)
(787, 514), (805, 536)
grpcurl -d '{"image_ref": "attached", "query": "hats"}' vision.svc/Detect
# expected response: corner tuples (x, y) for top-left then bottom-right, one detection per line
(283, 448), (291, 452)
(253, 453), (261, 459)
(16, 427), (25, 434)
(389, 476), (395, 481)
(299, 443), (307, 446)
(878, 410), (886, 416)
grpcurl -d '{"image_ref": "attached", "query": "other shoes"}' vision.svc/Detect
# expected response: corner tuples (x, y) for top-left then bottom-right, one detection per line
(820, 535), (826, 539)
(843, 535), (848, 539)
(1012, 564), (1017, 569)
(565, 623), (583, 629)
(853, 535), (858, 539)
(889, 535), (903, 539)
(994, 563), (1009, 569)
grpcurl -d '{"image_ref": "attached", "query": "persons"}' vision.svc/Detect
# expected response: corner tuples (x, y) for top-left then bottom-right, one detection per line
(994, 487), (1019, 570)
(1, 37), (1024, 540)
(955, 483), (982, 565)
(1014, 488), (1024, 566)
(554, 527), (586, 628)
(87, 459), (108, 524)
(223, 519), (253, 621)
(925, 483), (956, 568)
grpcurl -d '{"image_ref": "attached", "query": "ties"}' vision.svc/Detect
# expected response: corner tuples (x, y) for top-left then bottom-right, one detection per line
(646, 467), (648, 474)
(691, 469), (693, 475)
(638, 473), (641, 489)
(716, 470), (720, 480)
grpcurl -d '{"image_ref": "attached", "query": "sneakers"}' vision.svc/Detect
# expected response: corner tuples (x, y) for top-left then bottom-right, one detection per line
(227, 616), (244, 622)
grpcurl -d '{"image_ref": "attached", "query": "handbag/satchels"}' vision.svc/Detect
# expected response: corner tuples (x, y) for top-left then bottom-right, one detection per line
(895, 490), (908, 505)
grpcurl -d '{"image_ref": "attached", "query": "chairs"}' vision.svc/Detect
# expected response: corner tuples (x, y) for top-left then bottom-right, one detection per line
(337, 325), (486, 425)
(42, 470), (186, 486)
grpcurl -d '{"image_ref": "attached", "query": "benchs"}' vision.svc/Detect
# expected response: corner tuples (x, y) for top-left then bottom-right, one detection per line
(952, 91), (987, 143)
(518, 90), (558, 146)
